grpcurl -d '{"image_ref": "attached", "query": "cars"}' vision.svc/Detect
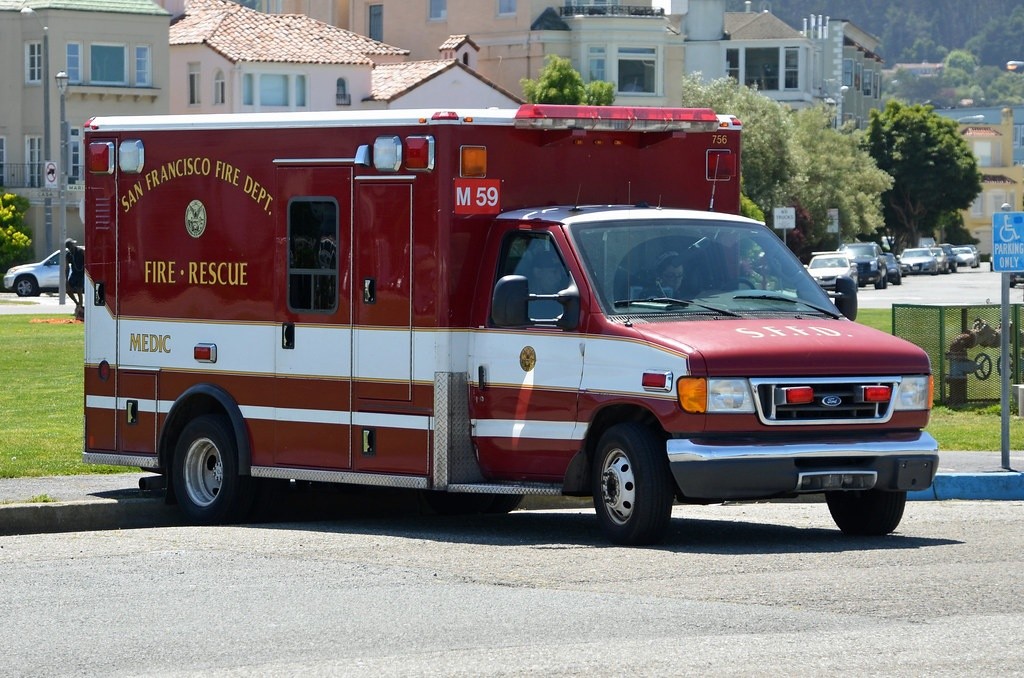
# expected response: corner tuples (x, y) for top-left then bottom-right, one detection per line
(884, 252), (902, 285)
(803, 251), (858, 292)
(3, 245), (85, 297)
(896, 243), (981, 277)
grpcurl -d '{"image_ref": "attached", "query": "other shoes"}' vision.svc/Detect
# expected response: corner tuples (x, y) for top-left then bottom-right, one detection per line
(75, 302), (83, 312)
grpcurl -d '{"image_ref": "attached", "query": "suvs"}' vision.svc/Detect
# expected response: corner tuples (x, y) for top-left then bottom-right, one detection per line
(836, 242), (888, 290)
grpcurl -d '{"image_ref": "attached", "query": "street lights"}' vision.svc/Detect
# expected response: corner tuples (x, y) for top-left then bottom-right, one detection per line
(55, 70), (70, 305)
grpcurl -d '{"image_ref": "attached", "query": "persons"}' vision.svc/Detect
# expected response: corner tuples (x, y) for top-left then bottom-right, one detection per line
(528, 251), (564, 320)
(64, 238), (85, 316)
(640, 255), (689, 300)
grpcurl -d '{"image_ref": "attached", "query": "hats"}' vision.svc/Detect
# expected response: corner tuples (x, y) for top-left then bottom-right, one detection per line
(533, 252), (561, 268)
(65, 237), (77, 247)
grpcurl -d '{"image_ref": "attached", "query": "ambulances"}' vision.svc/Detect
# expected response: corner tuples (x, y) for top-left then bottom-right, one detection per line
(80, 105), (940, 538)
